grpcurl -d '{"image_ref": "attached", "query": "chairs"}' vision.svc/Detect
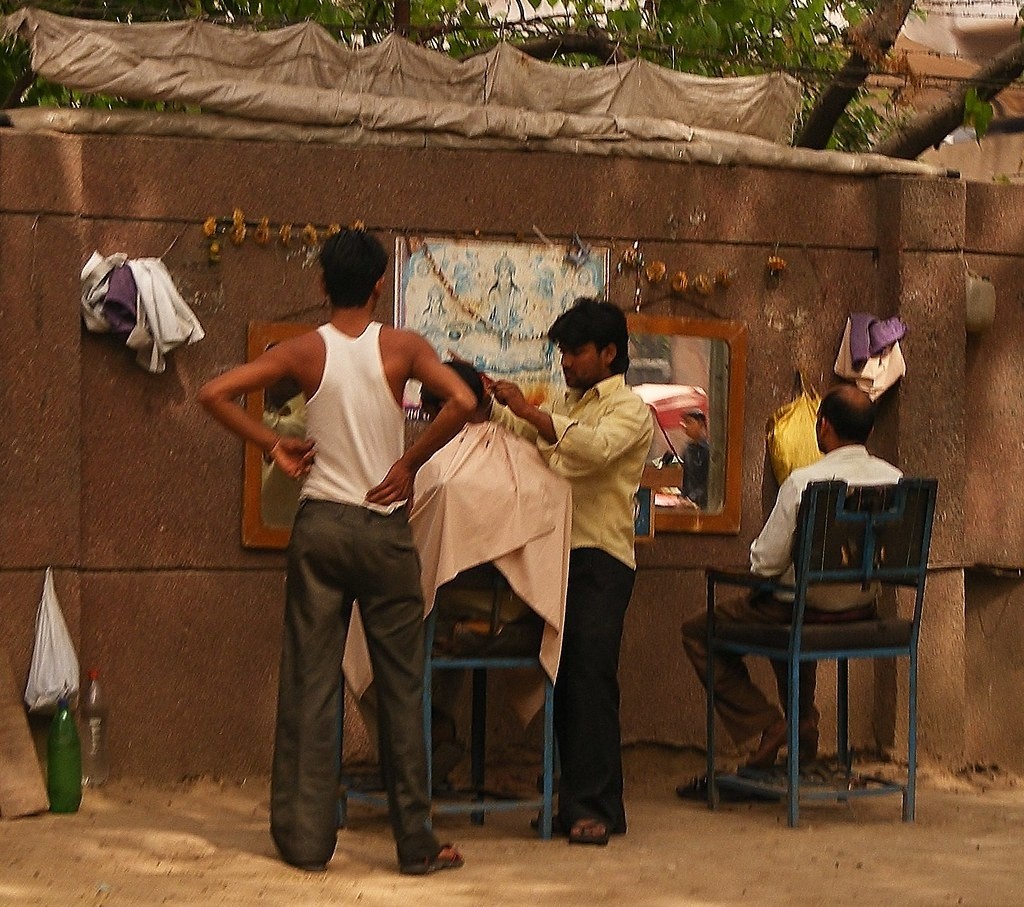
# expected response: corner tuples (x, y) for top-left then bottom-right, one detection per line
(699, 477), (940, 826)
(329, 480), (569, 840)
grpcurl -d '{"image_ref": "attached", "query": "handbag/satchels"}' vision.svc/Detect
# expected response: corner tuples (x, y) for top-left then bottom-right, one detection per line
(26, 566), (81, 714)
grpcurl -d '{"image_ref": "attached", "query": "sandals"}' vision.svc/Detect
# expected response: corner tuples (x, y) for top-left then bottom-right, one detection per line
(530, 815), (567, 836)
(569, 820), (609, 845)
(410, 846), (465, 875)
(304, 862), (328, 872)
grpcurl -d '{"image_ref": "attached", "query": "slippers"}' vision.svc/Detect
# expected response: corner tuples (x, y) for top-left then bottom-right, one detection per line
(679, 770), (788, 802)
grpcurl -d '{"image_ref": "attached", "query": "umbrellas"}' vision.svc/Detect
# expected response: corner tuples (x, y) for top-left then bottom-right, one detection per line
(632, 383), (707, 432)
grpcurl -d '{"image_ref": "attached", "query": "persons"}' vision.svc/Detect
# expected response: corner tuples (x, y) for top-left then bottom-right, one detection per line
(678, 408), (711, 509)
(338, 360), (573, 798)
(200, 232), (476, 877)
(484, 297), (657, 842)
(682, 384), (908, 773)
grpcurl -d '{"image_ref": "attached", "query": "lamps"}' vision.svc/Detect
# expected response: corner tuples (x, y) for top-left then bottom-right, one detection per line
(959, 266), (998, 330)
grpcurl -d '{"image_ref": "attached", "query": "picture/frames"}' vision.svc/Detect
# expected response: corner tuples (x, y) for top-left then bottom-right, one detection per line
(388, 234), (611, 429)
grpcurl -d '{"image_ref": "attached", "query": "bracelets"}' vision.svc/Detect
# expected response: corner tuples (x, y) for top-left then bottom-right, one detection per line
(270, 438), (279, 454)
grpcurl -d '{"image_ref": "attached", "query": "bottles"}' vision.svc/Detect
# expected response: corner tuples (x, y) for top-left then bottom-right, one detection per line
(46, 699), (83, 813)
(78, 673), (110, 786)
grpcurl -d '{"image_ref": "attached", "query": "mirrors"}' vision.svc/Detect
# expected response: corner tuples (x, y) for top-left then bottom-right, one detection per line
(625, 312), (745, 535)
(243, 320), (312, 550)
